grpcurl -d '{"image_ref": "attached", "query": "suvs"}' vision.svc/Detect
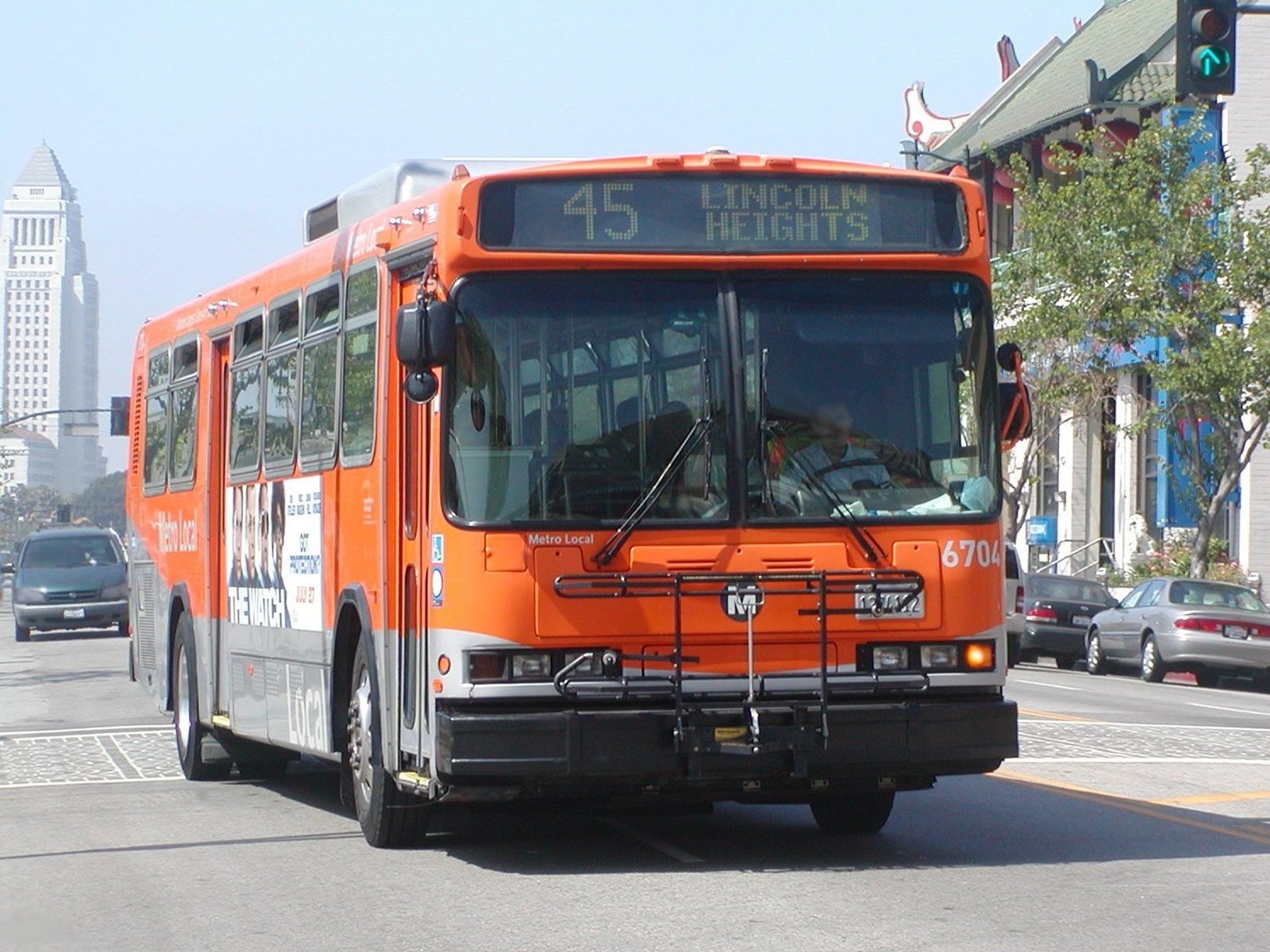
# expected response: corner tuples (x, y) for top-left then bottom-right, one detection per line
(1002, 541), (1027, 669)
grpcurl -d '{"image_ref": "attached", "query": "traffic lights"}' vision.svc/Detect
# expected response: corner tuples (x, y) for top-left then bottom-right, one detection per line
(1177, 0), (1237, 96)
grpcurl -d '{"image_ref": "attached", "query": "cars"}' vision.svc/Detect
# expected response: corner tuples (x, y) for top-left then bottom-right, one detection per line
(1023, 574), (1115, 670)
(1084, 576), (1270, 693)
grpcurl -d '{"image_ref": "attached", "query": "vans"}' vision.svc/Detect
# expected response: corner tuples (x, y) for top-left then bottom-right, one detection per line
(1, 523), (128, 642)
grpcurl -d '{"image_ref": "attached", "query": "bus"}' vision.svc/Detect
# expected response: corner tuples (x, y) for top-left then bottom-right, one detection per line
(127, 147), (1033, 851)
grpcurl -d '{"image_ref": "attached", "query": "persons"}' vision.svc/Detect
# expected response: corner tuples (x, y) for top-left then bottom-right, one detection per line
(779, 394), (895, 494)
(78, 542), (101, 566)
(224, 477), (294, 628)
(523, 397), (727, 493)
